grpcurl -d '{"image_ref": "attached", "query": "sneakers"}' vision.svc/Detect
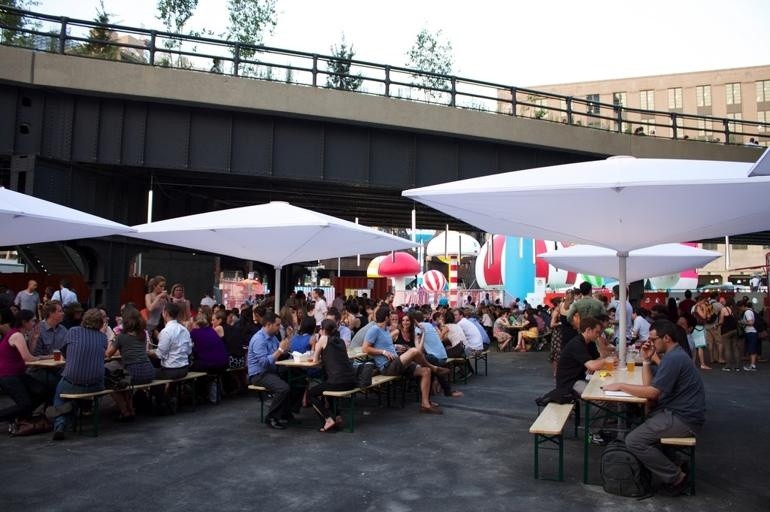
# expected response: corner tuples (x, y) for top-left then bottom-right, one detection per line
(589, 433), (607, 445)
(735, 368), (740, 372)
(701, 365), (712, 369)
(45, 402), (72, 417)
(53, 424), (65, 440)
(429, 400), (440, 407)
(722, 367), (731, 371)
(742, 364), (760, 371)
(449, 389), (463, 396)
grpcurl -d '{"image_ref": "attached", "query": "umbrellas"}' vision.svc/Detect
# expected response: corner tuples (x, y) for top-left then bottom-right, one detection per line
(1, 183), (139, 246)
(118, 201), (423, 317)
(402, 155), (770, 441)
(535, 243), (723, 286)
(747, 144), (769, 177)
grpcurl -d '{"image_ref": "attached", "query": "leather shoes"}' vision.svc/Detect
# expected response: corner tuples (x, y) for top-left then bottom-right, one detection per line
(431, 367), (451, 375)
(265, 417), (286, 429)
(419, 406), (443, 415)
(657, 474), (692, 497)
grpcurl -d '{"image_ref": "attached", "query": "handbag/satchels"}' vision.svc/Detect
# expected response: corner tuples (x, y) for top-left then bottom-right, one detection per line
(535, 388), (581, 426)
(692, 304), (706, 326)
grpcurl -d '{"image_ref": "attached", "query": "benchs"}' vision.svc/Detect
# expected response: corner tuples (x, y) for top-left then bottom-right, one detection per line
(446, 350), (490, 383)
(59, 371), (207, 437)
(322, 373), (401, 432)
(24, 354), (121, 411)
(529, 403), (579, 482)
(208, 367), (247, 405)
(660, 437), (696, 494)
(248, 384), (272, 424)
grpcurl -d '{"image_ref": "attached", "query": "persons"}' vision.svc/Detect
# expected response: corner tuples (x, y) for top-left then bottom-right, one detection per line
(106, 301), (155, 423)
(678, 289), (695, 317)
(96, 303), (115, 352)
(146, 272), (170, 335)
(44, 279), (79, 310)
(513, 309), (538, 352)
(43, 286), (54, 302)
(561, 119), (566, 124)
(412, 312), (447, 362)
(714, 296), (742, 373)
(405, 275), (419, 289)
(648, 290), (678, 330)
(493, 310), (512, 352)
(748, 275), (762, 294)
(115, 300), (125, 331)
(705, 291), (726, 365)
(734, 299), (763, 370)
(390, 313), (464, 407)
(226, 288), (345, 316)
(307, 319), (357, 431)
(556, 316), (622, 447)
(453, 309), (483, 358)
(9, 305), (21, 314)
(543, 304), (551, 334)
(148, 304), (192, 415)
(690, 296), (713, 370)
(464, 311), (490, 351)
(746, 138), (759, 148)
(475, 292), (499, 335)
(566, 281), (605, 333)
(549, 289), (574, 378)
(14, 279), (40, 321)
(248, 311), (297, 429)
(26, 301), (70, 386)
(280, 316), (320, 359)
(495, 297), (545, 318)
(634, 126), (646, 136)
(46, 308), (108, 440)
(458, 279), (465, 287)
(173, 283), (190, 320)
(712, 137), (720, 142)
(0, 309), (48, 438)
(607, 284), (632, 349)
(1, 285), (15, 325)
(350, 322), (378, 347)
(362, 302), (452, 414)
(683, 135), (688, 138)
(318, 309), (352, 348)
(342, 287), (446, 327)
(446, 293), (477, 320)
(440, 310), (473, 381)
(599, 319), (707, 497)
(577, 120), (581, 125)
(191, 290), (247, 371)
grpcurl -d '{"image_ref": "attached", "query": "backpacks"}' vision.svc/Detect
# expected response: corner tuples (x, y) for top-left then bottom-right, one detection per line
(703, 299), (717, 324)
(744, 307), (766, 333)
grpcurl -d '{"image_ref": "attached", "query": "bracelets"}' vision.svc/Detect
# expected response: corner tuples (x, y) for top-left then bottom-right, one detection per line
(382, 348), (386, 357)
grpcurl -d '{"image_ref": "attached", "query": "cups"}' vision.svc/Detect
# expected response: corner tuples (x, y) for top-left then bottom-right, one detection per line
(53, 350), (61, 362)
(627, 359), (635, 372)
(606, 358), (614, 372)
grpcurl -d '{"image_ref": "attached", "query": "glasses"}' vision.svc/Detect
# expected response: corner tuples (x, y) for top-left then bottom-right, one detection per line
(648, 336), (660, 342)
(319, 328), (323, 329)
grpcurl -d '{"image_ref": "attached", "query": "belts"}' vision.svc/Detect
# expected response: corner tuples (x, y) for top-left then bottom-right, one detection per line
(61, 376), (102, 388)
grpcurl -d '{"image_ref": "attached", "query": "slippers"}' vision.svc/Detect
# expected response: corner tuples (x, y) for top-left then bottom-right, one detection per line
(319, 427), (337, 433)
(498, 343), (504, 353)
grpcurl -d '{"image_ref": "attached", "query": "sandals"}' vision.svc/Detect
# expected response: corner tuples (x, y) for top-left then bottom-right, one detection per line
(112, 407), (137, 424)
(514, 344), (521, 351)
(519, 347), (527, 352)
(169, 397), (177, 415)
